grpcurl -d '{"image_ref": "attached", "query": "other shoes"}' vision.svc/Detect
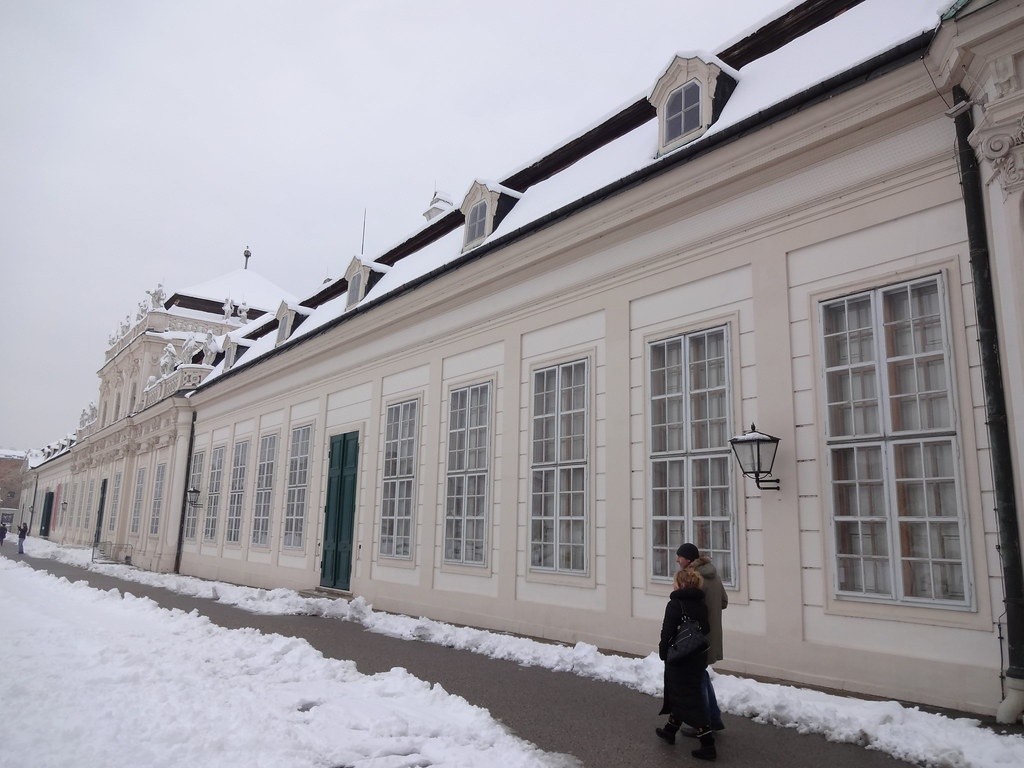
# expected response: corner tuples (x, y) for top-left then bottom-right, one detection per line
(681, 727), (698, 737)
(711, 721), (724, 731)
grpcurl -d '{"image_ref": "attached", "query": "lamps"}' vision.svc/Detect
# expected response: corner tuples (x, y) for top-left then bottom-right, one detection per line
(186, 487), (204, 508)
(61, 502), (68, 512)
(29, 506), (34, 513)
(726, 422), (782, 493)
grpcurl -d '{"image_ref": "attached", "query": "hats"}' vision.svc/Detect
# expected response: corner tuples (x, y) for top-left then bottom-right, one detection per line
(676, 543), (700, 560)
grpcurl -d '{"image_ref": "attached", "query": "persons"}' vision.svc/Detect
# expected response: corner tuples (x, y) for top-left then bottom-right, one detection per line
(675, 543), (730, 731)
(80, 401), (96, 426)
(181, 332), (200, 364)
(159, 343), (178, 375)
(0, 524), (7, 546)
(17, 523), (28, 554)
(202, 329), (219, 366)
(237, 300), (250, 323)
(221, 293), (235, 318)
(108, 283), (166, 347)
(655, 569), (727, 762)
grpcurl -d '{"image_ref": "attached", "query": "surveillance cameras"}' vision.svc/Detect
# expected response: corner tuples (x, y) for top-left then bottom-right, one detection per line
(945, 100), (973, 118)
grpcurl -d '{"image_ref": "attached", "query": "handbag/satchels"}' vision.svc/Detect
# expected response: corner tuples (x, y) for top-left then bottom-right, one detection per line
(666, 598), (711, 665)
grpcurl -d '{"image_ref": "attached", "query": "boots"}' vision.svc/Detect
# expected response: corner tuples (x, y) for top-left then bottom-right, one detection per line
(691, 725), (717, 761)
(655, 715), (682, 744)
